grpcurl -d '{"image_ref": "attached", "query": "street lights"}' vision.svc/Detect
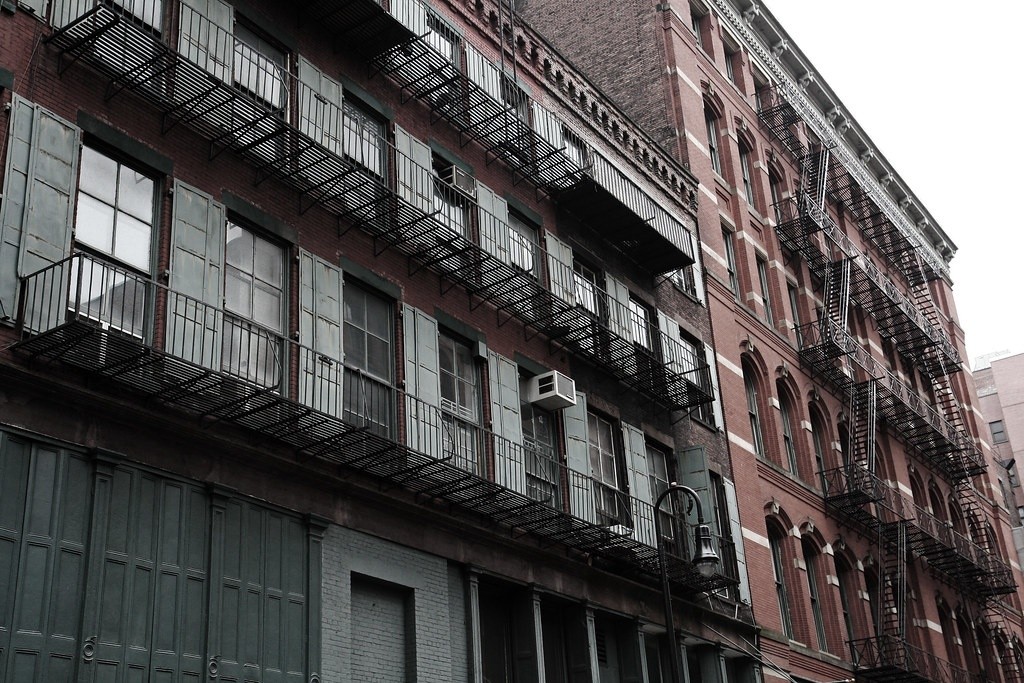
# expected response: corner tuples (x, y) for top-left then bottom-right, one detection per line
(655, 485), (723, 683)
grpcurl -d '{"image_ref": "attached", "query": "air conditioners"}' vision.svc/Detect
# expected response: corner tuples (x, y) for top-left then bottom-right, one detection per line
(530, 368), (578, 409)
(442, 164), (477, 199)
(604, 523), (636, 548)
